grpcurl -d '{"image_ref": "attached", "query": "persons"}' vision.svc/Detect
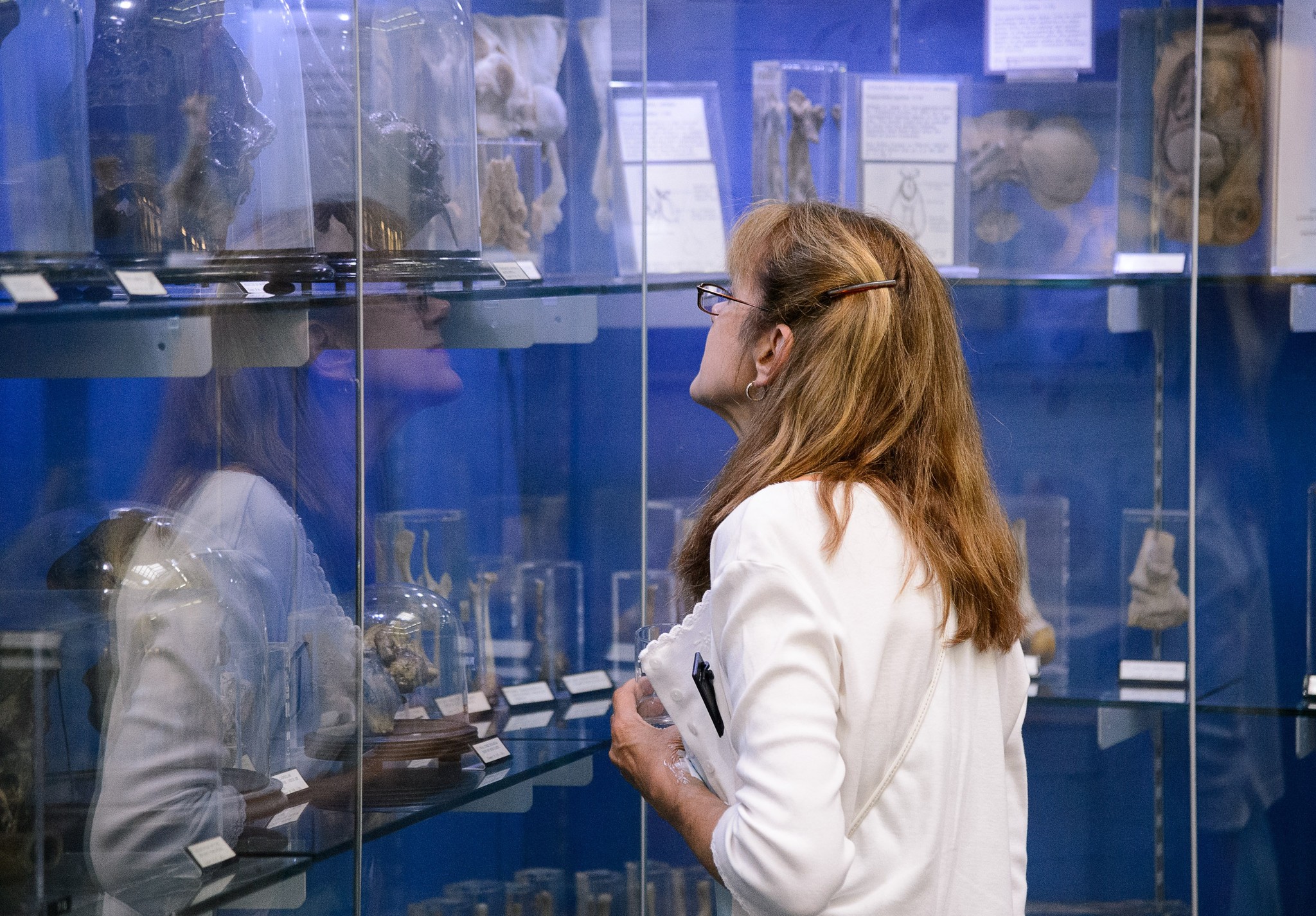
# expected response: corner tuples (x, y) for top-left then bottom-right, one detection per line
(87, 197), (465, 916)
(608, 198), (1029, 916)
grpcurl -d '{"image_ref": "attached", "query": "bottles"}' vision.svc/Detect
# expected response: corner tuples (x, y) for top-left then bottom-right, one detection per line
(0, 631), (72, 916)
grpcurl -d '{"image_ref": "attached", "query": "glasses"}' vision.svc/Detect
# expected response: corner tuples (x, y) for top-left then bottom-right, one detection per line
(383, 281), (434, 312)
(697, 282), (774, 315)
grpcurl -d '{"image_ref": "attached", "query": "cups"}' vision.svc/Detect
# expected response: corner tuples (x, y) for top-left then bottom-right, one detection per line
(634, 623), (675, 728)
